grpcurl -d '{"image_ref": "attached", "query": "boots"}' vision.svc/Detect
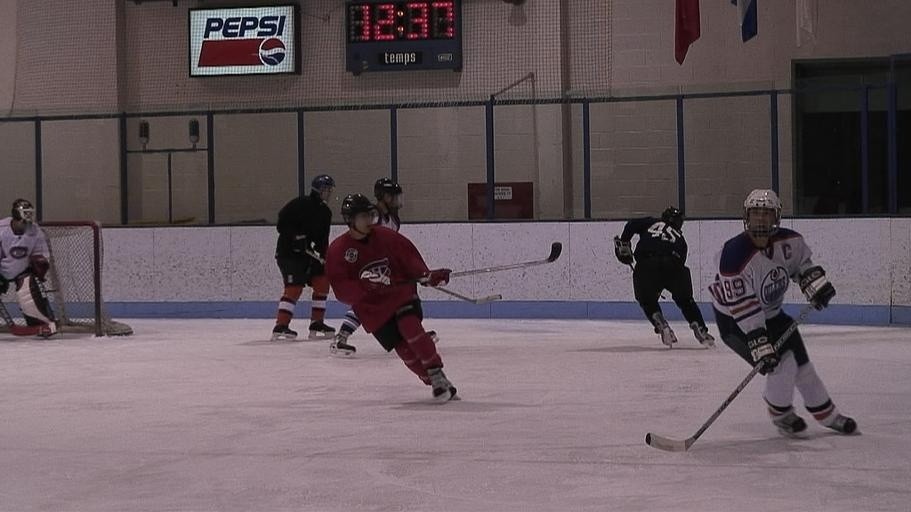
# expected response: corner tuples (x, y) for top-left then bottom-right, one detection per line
(332, 331), (355, 353)
(653, 312), (678, 344)
(690, 320), (716, 344)
(427, 368), (457, 404)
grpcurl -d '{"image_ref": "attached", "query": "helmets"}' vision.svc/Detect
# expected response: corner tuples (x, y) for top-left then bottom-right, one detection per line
(311, 174), (336, 204)
(660, 207), (683, 229)
(342, 194), (376, 228)
(743, 189), (782, 238)
(374, 177), (401, 205)
(11, 198), (34, 221)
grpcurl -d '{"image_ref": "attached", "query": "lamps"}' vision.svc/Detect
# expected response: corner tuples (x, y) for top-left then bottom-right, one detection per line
(124, 118), (209, 226)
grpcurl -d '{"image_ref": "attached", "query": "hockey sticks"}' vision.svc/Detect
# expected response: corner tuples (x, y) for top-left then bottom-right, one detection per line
(393, 242), (561, 285)
(645, 303), (814, 452)
(0, 299), (47, 335)
(433, 285), (501, 306)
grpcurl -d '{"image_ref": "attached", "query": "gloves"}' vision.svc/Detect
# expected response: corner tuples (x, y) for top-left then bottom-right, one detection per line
(743, 327), (779, 376)
(613, 235), (634, 265)
(298, 236), (309, 252)
(423, 266), (451, 289)
(800, 265), (836, 312)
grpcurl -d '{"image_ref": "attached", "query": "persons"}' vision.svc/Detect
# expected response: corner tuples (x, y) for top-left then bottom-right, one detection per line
(613, 207), (715, 346)
(0, 198), (62, 341)
(708, 188), (856, 434)
(329, 177), (403, 354)
(271, 174), (337, 338)
(324, 192), (458, 401)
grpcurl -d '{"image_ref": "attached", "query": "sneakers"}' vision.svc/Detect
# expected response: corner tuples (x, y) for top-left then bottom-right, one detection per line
(273, 325), (297, 337)
(771, 413), (808, 434)
(308, 321), (336, 332)
(826, 414), (857, 433)
